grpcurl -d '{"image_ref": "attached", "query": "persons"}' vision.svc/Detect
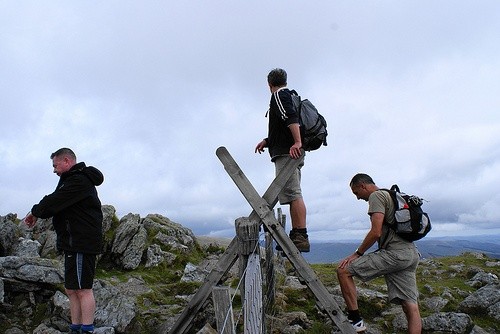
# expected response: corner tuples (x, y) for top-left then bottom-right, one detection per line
(336, 173), (421, 334)
(23, 148), (104, 334)
(255, 68), (311, 257)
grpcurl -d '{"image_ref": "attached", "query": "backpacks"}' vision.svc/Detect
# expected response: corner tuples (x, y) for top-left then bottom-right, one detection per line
(265, 89), (327, 151)
(374, 184), (432, 241)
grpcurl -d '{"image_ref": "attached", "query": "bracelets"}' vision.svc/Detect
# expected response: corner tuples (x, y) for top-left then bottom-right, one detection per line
(354, 248), (363, 256)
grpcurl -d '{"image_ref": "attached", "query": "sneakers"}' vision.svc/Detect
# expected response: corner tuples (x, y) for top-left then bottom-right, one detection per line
(346, 319), (365, 332)
(275, 233), (310, 257)
